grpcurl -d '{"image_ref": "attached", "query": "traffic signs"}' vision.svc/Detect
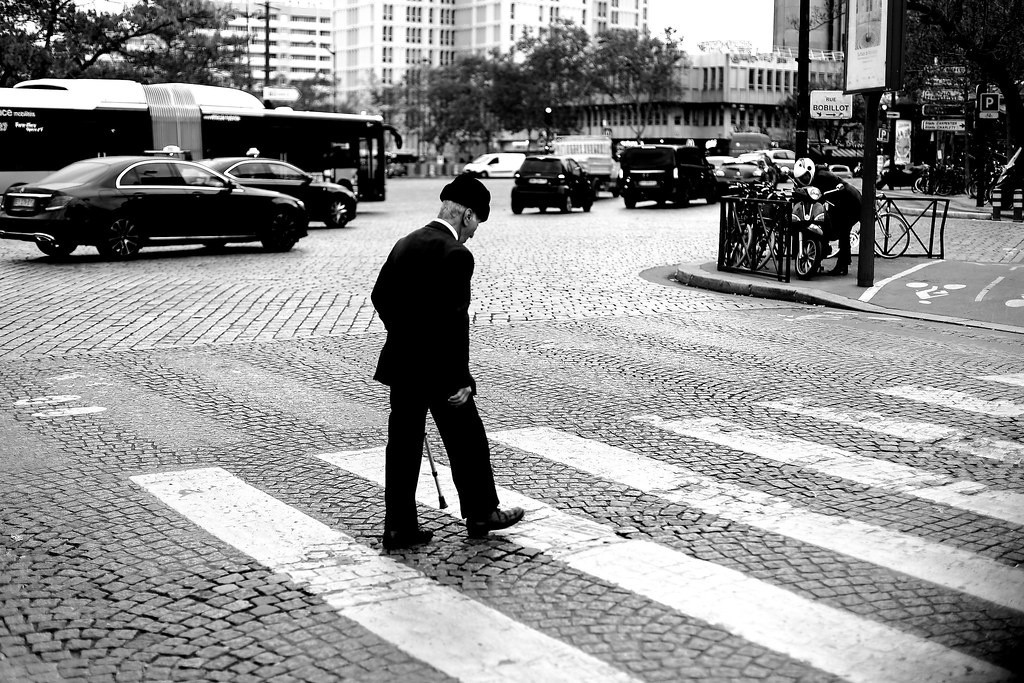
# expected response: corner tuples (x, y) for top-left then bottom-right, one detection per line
(922, 119), (966, 132)
(922, 104), (966, 117)
(262, 86), (304, 102)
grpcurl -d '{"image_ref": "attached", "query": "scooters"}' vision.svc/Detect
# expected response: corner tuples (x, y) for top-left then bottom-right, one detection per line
(780, 165), (845, 281)
(876, 157), (930, 191)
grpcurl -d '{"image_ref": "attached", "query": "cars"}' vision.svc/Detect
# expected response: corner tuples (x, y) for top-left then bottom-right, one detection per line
(180, 156), (360, 230)
(706, 147), (796, 181)
(714, 156), (777, 199)
(511, 154), (595, 214)
(0, 155), (310, 262)
(827, 164), (854, 179)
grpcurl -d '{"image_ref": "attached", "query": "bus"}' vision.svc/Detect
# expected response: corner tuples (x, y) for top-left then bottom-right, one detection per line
(729, 132), (772, 159)
(0, 78), (404, 203)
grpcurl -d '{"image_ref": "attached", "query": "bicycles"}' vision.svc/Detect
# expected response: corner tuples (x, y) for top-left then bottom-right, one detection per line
(912, 139), (1008, 205)
(823, 189), (909, 259)
(721, 181), (793, 274)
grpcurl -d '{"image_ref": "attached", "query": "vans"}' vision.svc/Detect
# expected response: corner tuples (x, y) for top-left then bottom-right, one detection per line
(461, 152), (526, 179)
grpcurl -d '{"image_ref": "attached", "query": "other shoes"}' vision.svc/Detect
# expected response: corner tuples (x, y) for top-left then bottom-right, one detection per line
(826, 262), (848, 275)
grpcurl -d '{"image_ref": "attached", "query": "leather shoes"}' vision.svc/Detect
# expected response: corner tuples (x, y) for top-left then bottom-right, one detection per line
(465, 507), (524, 537)
(382, 524), (434, 550)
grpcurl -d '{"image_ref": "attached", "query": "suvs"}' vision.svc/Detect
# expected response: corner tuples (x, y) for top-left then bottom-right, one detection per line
(621, 144), (720, 209)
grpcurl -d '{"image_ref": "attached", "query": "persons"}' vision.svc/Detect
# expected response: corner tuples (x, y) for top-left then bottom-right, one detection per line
(370, 175), (527, 551)
(791, 157), (862, 278)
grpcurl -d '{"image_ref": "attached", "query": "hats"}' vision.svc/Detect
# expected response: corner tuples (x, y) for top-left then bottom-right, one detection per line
(440, 174), (491, 222)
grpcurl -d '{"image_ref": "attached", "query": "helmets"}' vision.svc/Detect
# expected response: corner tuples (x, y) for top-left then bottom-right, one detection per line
(794, 157), (815, 187)
